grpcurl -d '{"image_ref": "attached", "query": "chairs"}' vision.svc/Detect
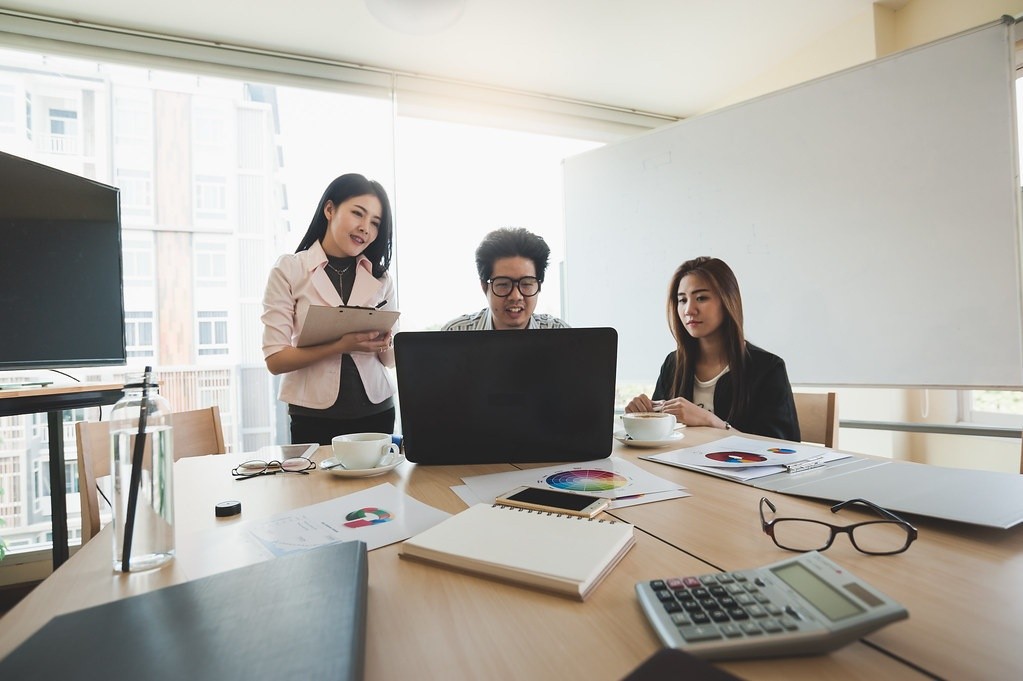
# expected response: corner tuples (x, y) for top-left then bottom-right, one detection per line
(791, 392), (840, 450)
(77, 405), (225, 552)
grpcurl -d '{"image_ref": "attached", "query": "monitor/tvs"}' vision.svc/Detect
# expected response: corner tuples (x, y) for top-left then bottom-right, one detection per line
(0, 151), (127, 372)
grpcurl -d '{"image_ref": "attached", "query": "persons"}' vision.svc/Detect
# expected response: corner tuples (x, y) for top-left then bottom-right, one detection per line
(441, 227), (570, 330)
(625, 256), (802, 443)
(260, 173), (396, 446)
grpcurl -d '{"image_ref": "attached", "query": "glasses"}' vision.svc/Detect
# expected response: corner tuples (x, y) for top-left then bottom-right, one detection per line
(759, 497), (919, 556)
(486, 276), (542, 296)
(231, 456), (316, 481)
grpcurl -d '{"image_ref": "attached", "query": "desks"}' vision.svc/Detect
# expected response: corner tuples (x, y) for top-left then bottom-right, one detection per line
(0, 384), (127, 574)
(0, 417), (1023, 681)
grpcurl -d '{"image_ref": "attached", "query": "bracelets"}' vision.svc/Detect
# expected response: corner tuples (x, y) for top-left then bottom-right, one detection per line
(383, 334), (394, 352)
(725, 423), (731, 430)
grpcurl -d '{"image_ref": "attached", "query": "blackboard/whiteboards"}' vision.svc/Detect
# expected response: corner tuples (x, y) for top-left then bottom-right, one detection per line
(561, 14), (1023, 392)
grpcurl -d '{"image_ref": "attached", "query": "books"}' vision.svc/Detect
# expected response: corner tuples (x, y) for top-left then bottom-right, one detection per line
(397, 503), (636, 601)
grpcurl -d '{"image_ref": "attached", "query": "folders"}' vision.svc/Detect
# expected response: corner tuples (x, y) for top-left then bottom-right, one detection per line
(637, 435), (1023, 529)
(0, 540), (367, 681)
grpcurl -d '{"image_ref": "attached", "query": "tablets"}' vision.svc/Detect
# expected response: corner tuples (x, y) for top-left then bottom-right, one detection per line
(239, 443), (320, 468)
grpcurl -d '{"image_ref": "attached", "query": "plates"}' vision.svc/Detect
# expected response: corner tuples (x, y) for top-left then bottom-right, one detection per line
(320, 452), (406, 478)
(613, 429), (685, 448)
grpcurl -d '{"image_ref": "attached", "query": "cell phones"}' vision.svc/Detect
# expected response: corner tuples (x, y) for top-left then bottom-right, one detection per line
(495, 486), (612, 518)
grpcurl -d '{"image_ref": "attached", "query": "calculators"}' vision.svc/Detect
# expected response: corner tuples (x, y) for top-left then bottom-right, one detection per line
(634, 550), (910, 662)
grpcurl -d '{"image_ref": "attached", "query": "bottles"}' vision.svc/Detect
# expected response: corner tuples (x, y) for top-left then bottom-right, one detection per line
(108, 370), (175, 573)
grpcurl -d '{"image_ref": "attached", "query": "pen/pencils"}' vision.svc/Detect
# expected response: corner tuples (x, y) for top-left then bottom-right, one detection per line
(375, 300), (387, 308)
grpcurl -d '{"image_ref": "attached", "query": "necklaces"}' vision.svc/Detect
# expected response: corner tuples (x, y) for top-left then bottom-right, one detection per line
(328, 264), (348, 275)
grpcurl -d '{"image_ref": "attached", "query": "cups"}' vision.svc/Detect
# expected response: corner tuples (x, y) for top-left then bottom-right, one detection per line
(619, 411), (677, 441)
(332, 432), (400, 471)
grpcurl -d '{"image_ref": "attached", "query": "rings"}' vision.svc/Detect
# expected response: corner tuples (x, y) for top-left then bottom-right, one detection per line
(380, 347), (382, 352)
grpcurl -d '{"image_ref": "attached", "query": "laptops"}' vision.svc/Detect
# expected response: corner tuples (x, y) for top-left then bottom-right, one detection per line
(393, 327), (618, 465)
